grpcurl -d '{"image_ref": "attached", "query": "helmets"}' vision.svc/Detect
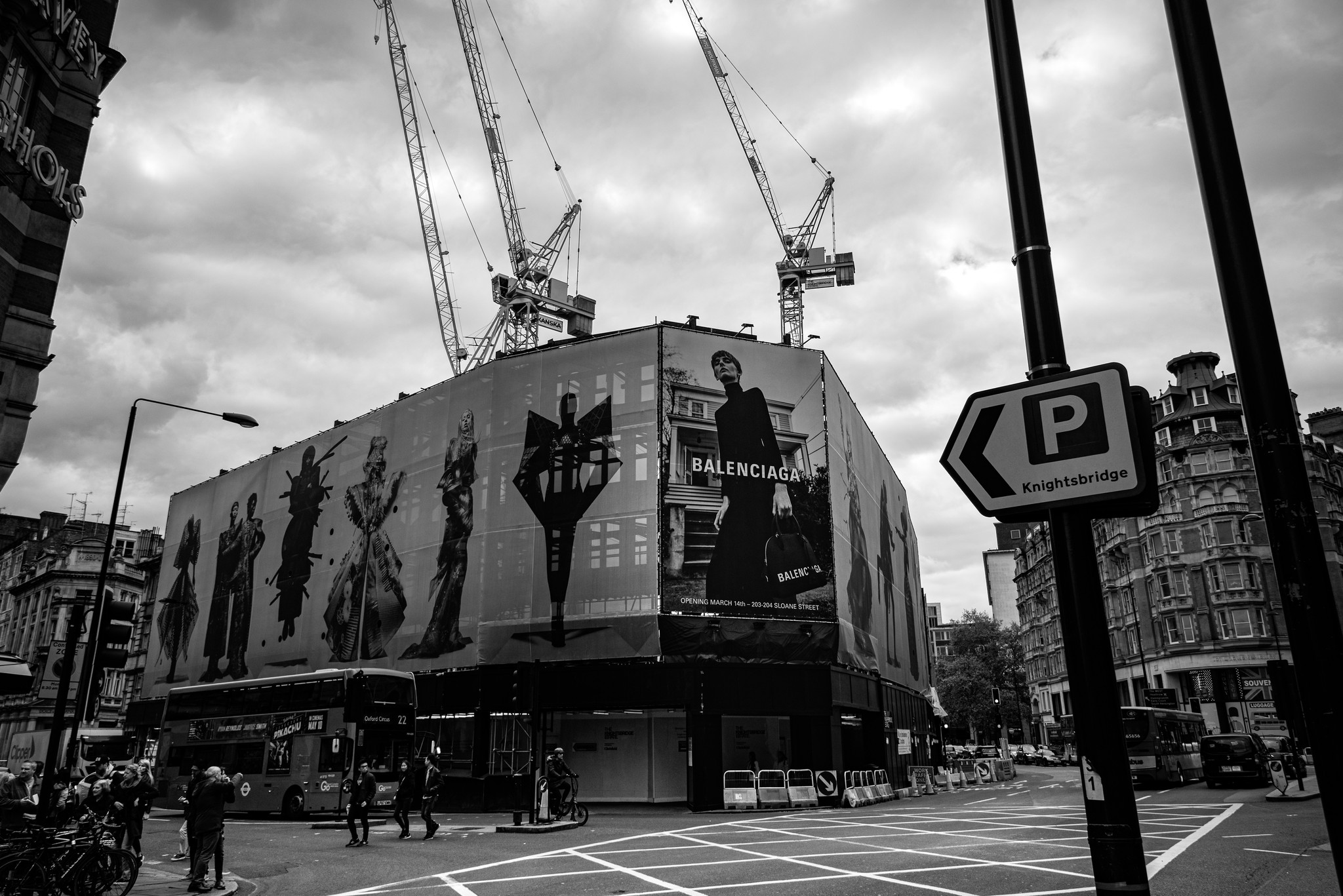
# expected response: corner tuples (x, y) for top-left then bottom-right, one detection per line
(554, 747), (564, 758)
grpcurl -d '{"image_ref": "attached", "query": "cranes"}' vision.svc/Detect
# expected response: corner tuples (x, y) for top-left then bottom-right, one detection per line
(678, 3), (859, 347)
(376, 0), (588, 376)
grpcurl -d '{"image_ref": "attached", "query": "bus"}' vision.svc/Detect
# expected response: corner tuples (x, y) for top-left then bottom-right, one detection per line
(147, 667), (419, 821)
(1060, 714), (1078, 766)
(1121, 703), (1206, 785)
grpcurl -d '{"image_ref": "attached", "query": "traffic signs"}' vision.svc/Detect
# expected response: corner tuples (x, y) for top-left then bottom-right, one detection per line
(1143, 688), (1178, 711)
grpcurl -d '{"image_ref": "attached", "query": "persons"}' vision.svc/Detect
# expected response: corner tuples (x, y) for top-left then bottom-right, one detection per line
(705, 350), (799, 612)
(199, 492), (266, 684)
(171, 762), (244, 893)
(397, 408), (481, 662)
(393, 759), (416, 839)
(323, 437), (407, 662)
(0, 755), (156, 881)
(421, 753), (444, 840)
(546, 747), (580, 821)
(345, 759), (376, 847)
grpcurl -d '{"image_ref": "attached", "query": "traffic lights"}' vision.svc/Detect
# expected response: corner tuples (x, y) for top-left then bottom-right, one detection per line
(96, 591), (137, 669)
(991, 687), (1001, 705)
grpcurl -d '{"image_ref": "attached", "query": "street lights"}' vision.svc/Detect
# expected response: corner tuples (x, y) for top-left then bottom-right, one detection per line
(65, 396), (256, 802)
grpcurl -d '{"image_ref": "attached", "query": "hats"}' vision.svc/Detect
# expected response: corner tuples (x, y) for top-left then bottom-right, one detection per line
(90, 753), (110, 766)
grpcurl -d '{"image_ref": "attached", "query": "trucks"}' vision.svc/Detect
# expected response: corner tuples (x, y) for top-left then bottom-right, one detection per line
(8, 728), (135, 803)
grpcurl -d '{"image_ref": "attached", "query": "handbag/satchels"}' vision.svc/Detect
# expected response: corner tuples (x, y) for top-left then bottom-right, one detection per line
(393, 789), (398, 800)
(766, 509), (828, 597)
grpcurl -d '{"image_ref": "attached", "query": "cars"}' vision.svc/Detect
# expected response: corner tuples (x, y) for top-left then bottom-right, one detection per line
(942, 743), (1015, 763)
(1195, 734), (1269, 790)
(1259, 734), (1295, 782)
(1034, 749), (1066, 766)
(1016, 744), (1042, 765)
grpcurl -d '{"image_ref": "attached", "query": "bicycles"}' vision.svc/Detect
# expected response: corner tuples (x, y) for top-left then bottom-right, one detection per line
(0, 804), (139, 896)
(549, 773), (589, 827)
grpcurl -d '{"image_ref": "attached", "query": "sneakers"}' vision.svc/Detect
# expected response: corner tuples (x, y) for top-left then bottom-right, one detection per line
(422, 832), (433, 840)
(187, 879), (213, 893)
(560, 801), (569, 807)
(136, 853), (144, 868)
(357, 840), (369, 847)
(204, 874), (210, 880)
(432, 822), (440, 836)
(170, 854), (190, 860)
(179, 873), (193, 881)
(345, 838), (360, 847)
(214, 880), (225, 890)
(399, 833), (411, 839)
(113, 877), (130, 885)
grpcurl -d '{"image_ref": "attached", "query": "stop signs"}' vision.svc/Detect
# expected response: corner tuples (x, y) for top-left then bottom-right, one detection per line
(1009, 728), (1014, 734)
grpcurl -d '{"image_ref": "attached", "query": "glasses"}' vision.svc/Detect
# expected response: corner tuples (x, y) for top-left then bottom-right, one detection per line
(360, 765), (367, 768)
